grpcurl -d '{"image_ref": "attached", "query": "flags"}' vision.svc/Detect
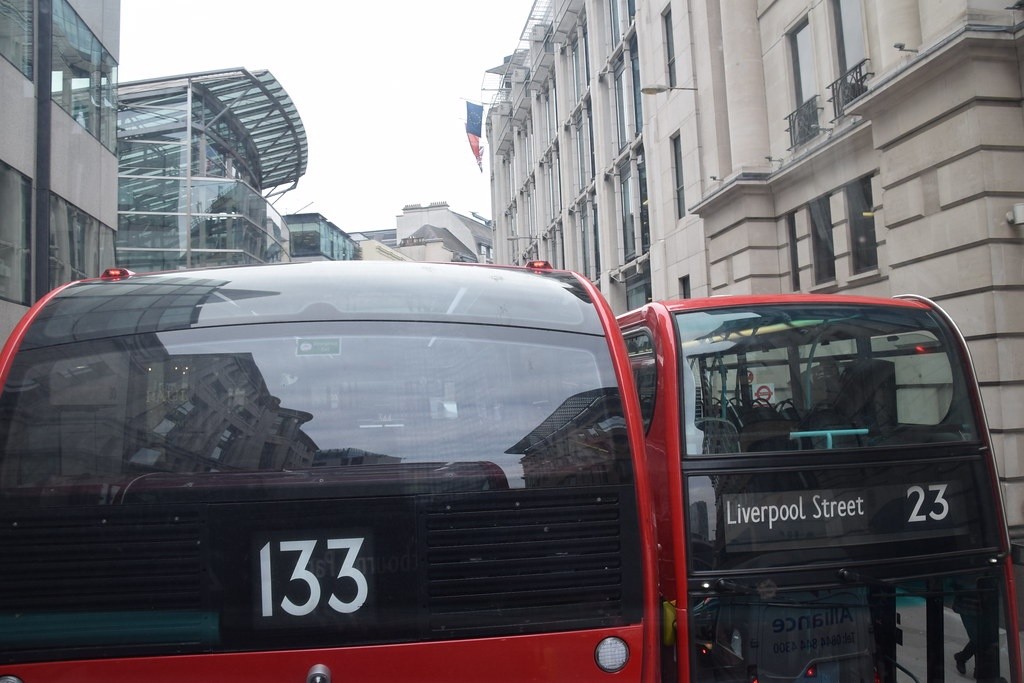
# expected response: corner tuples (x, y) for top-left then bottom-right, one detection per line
(475, 146), (484, 173)
(465, 124), (481, 160)
(466, 101), (483, 138)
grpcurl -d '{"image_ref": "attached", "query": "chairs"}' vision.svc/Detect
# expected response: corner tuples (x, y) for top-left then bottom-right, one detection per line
(697, 397), (863, 451)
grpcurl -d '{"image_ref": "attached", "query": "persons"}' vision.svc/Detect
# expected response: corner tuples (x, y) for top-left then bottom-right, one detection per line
(952, 596), (978, 679)
(843, 373), (890, 429)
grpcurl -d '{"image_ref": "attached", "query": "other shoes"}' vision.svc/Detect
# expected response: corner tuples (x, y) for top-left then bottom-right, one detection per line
(954, 653), (966, 674)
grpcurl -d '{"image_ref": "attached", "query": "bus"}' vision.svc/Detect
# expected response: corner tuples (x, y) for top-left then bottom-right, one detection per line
(1, 260), (658, 683)
(612, 289), (1024, 683)
(0, 457), (508, 516)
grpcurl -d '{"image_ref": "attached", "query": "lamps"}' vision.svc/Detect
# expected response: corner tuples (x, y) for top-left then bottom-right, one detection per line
(507, 237), (540, 241)
(811, 124), (834, 132)
(894, 43), (919, 56)
(641, 87), (698, 95)
(710, 176), (725, 182)
(765, 156), (783, 162)
